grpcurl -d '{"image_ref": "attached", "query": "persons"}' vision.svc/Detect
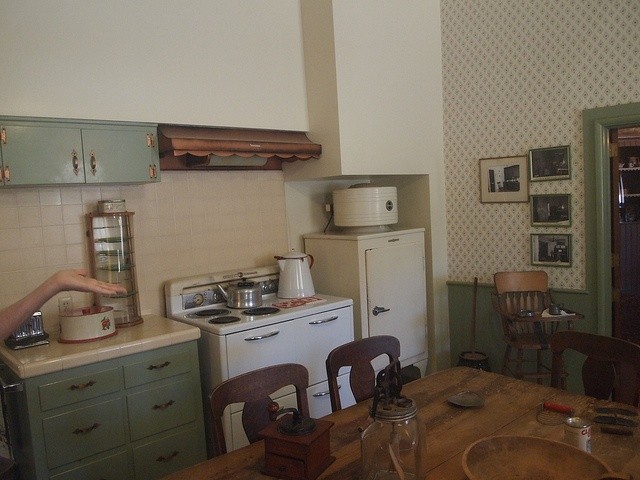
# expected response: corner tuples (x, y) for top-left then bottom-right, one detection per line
(1, 268), (128, 340)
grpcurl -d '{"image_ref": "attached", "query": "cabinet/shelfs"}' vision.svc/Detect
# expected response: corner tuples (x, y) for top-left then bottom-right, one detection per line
(220, 371), (357, 456)
(1, 115), (161, 187)
(1, 315), (208, 479)
(201, 298), (353, 415)
(304, 227), (429, 377)
(618, 143), (639, 224)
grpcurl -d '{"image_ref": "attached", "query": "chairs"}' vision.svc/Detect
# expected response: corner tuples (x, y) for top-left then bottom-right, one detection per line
(324, 334), (401, 414)
(210, 363), (311, 455)
(492, 270), (584, 385)
(546, 329), (640, 408)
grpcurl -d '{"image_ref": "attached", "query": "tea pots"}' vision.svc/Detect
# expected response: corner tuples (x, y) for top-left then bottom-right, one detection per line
(274, 247), (316, 298)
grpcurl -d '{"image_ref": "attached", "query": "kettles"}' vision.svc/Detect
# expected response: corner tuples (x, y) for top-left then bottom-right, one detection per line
(216, 277), (263, 309)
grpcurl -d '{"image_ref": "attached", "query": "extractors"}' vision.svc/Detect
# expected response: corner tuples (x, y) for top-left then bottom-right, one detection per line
(156, 123), (323, 171)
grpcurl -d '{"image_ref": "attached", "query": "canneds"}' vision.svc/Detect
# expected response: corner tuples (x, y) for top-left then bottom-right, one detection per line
(565, 417), (593, 454)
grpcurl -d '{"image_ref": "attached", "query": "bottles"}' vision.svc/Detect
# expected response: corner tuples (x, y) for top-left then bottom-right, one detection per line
(360, 399), (422, 480)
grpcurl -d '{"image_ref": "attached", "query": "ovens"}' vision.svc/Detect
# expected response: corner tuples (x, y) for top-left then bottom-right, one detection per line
(223, 305), (363, 454)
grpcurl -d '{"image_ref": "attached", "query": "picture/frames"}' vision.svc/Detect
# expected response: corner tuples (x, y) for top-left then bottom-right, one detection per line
(479, 155), (530, 203)
(529, 232), (572, 267)
(528, 145), (573, 182)
(528, 194), (571, 228)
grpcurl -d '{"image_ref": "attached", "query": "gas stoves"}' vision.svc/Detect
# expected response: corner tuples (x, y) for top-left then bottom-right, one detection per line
(193, 306), (280, 324)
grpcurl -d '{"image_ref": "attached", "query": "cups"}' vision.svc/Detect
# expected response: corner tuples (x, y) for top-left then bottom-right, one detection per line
(548, 303), (564, 315)
